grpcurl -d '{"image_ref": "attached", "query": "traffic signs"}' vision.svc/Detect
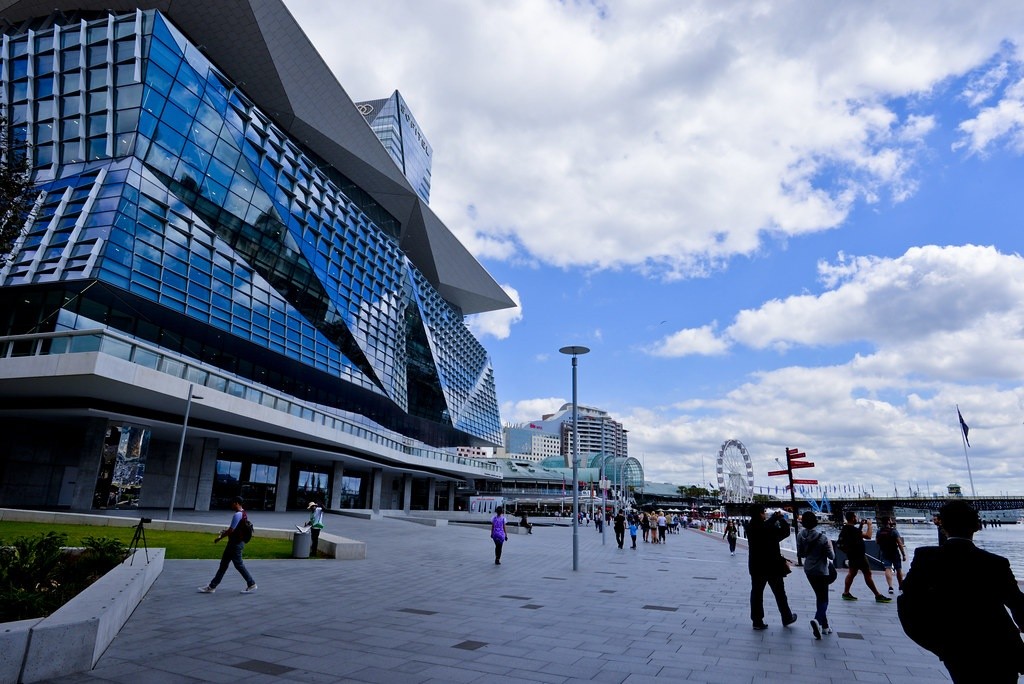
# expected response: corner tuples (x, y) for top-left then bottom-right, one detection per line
(790, 461), (809, 465)
(768, 470), (789, 476)
(792, 479), (818, 484)
(789, 453), (806, 459)
(788, 448), (798, 454)
(785, 485), (791, 489)
(790, 463), (815, 469)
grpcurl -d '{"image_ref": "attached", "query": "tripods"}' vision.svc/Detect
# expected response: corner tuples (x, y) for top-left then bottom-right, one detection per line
(121, 517), (152, 566)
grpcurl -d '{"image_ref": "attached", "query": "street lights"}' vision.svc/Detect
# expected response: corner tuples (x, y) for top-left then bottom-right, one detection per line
(167, 384), (204, 520)
(558, 344), (591, 572)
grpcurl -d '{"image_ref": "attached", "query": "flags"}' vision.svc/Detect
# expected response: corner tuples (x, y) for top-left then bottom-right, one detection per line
(958, 409), (971, 447)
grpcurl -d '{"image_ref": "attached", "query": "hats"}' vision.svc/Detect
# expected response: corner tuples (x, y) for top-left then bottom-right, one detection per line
(307, 502), (318, 509)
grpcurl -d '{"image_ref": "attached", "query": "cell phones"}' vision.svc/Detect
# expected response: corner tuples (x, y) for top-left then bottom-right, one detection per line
(771, 512), (777, 523)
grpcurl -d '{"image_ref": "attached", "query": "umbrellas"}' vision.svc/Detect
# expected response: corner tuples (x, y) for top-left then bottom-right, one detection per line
(713, 509), (721, 512)
(764, 508), (789, 514)
(656, 508), (700, 512)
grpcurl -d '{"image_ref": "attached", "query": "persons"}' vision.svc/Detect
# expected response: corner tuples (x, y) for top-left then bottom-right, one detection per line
(837, 511), (906, 603)
(198, 496), (258, 594)
(627, 509), (643, 529)
(664, 512), (672, 534)
(706, 519), (713, 534)
(671, 513), (679, 535)
(606, 511), (611, 527)
(896, 500), (1024, 684)
(747, 503), (797, 630)
(555, 508), (571, 517)
(680, 513), (705, 527)
(797, 511), (836, 640)
(513, 507), (523, 517)
(723, 520), (737, 556)
(738, 519), (748, 538)
(642, 512), (667, 544)
(304, 502), (323, 556)
(614, 509), (638, 549)
(491, 505), (508, 565)
(724, 521), (727, 532)
(577, 510), (603, 533)
(520, 512), (532, 535)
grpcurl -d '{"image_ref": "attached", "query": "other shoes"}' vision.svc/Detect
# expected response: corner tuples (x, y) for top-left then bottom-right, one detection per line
(198, 585), (215, 592)
(810, 619), (822, 640)
(731, 551), (735, 556)
(753, 623), (768, 630)
(822, 628), (832, 634)
(528, 532), (532, 534)
(888, 589), (894, 593)
(243, 584), (256, 593)
(783, 614), (797, 625)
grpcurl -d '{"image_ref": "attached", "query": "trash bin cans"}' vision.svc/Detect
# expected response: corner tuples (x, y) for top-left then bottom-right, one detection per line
(292, 531), (311, 558)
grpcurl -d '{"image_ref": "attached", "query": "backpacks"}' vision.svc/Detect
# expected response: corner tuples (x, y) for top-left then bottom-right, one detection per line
(234, 515), (254, 544)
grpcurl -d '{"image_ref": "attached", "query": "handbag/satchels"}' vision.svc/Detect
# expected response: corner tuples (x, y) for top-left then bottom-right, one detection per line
(826, 561), (837, 585)
(731, 532), (737, 538)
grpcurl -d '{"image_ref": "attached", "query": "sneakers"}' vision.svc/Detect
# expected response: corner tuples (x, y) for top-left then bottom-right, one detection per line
(875, 593), (892, 602)
(842, 592), (858, 600)
